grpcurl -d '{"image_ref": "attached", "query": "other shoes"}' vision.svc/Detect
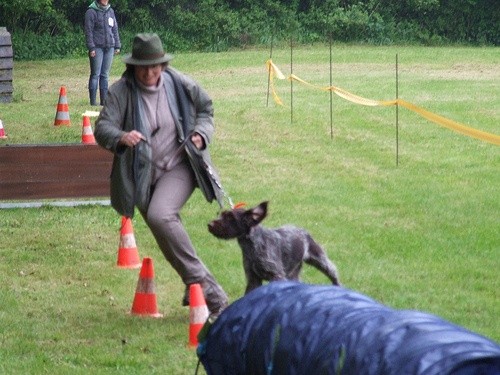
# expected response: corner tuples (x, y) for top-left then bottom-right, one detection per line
(92, 102), (98, 106)
(211, 303), (228, 314)
(183, 285), (189, 305)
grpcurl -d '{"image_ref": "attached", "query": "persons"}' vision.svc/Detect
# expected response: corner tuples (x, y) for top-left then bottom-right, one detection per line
(84, 0), (121, 106)
(94, 34), (228, 313)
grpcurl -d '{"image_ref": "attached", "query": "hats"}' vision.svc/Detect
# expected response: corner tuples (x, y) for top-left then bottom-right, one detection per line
(122, 33), (172, 65)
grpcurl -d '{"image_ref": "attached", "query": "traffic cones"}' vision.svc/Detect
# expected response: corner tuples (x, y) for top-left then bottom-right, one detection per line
(0, 120), (8, 139)
(82, 115), (96, 144)
(129, 256), (163, 318)
(187, 283), (211, 344)
(54, 87), (70, 125)
(116, 213), (141, 267)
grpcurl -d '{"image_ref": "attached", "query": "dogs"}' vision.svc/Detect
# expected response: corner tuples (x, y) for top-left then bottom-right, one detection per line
(207, 200), (340, 297)
